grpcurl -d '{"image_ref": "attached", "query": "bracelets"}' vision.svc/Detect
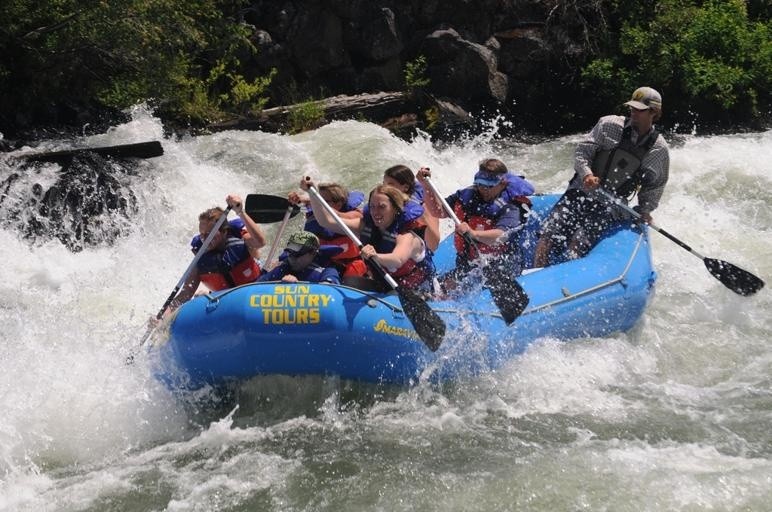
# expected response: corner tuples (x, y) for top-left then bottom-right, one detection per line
(236, 208), (244, 217)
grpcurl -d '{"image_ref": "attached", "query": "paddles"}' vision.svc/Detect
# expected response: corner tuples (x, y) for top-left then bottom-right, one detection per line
(591, 181), (765, 298)
(423, 168), (529, 326)
(245, 194), (312, 225)
(304, 177), (445, 352)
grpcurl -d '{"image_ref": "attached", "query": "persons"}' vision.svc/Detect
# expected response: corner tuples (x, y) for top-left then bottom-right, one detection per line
(297, 175), (437, 294)
(533, 85), (672, 267)
(252, 230), (342, 285)
(381, 164), (441, 255)
(414, 157), (537, 294)
(288, 179), (368, 287)
(146, 195), (265, 330)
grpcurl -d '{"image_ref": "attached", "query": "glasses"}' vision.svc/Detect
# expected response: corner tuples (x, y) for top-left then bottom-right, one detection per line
(283, 249), (312, 259)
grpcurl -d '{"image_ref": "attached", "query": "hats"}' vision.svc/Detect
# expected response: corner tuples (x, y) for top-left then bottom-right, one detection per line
(473, 169), (508, 188)
(282, 230), (321, 257)
(623, 87), (662, 111)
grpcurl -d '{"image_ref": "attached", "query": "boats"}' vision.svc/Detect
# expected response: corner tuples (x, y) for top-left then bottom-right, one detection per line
(138, 188), (654, 417)
